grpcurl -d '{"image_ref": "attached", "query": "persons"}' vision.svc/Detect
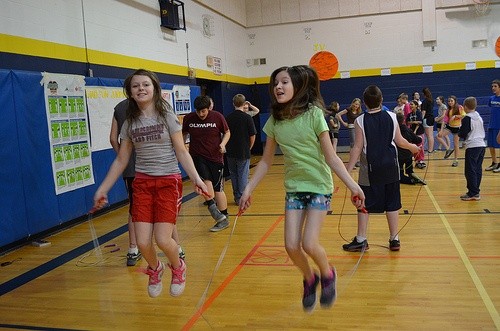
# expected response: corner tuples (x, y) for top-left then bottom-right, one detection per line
(483, 80), (500, 173)
(457, 97), (487, 202)
(203, 98), (219, 206)
(237, 67), (366, 311)
(109, 77), (186, 267)
(182, 96), (231, 232)
(225, 94), (257, 206)
(386, 89), (436, 169)
(434, 97), (451, 152)
(395, 114), (423, 183)
(327, 100), (340, 151)
(343, 86), (421, 251)
(243, 101), (259, 118)
(338, 98), (363, 171)
(436, 96), (465, 167)
(93, 69), (210, 298)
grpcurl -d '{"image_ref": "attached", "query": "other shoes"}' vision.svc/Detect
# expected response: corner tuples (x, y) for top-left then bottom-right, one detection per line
(427, 150), (433, 156)
(415, 162), (426, 169)
(444, 148), (454, 159)
(234, 197), (240, 205)
(452, 161), (457, 166)
(353, 166), (357, 169)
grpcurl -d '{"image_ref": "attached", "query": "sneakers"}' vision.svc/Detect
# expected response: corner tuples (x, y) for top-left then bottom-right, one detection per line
(460, 193), (480, 201)
(178, 250), (185, 260)
(126, 251), (142, 265)
(320, 265), (335, 308)
(485, 162), (496, 171)
(389, 239), (399, 250)
(209, 220), (229, 231)
(139, 260), (165, 298)
(342, 236), (369, 251)
(166, 259), (186, 296)
(302, 273), (319, 311)
(493, 163), (500, 172)
